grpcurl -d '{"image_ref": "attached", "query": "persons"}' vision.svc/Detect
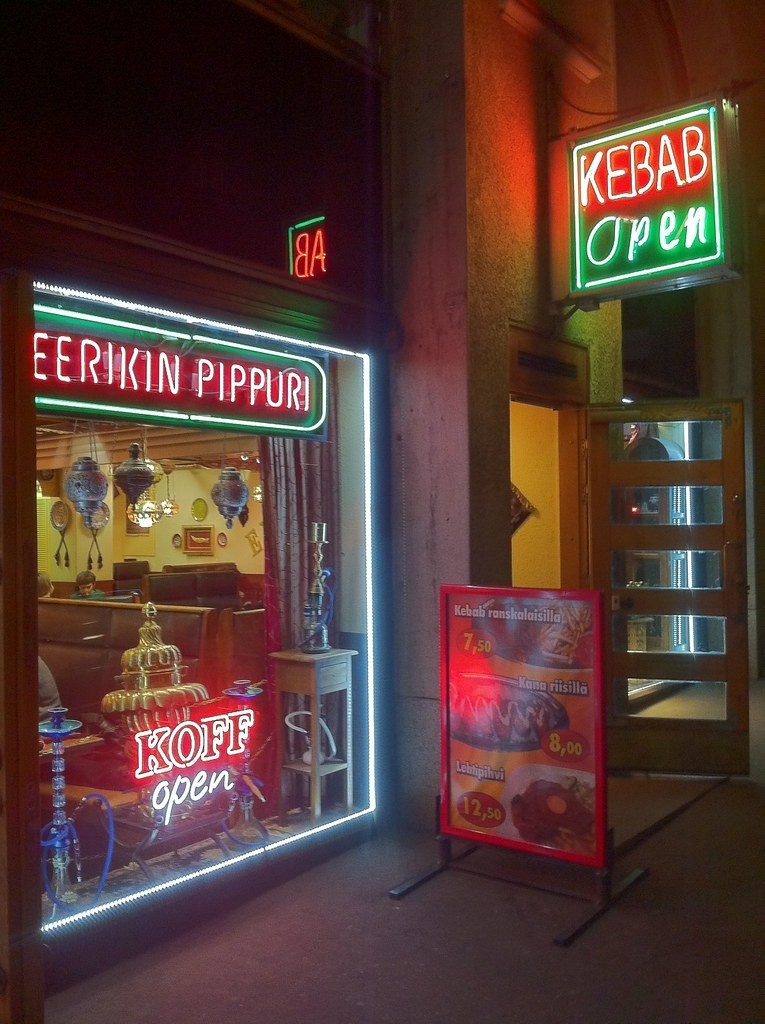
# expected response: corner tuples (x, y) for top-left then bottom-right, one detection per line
(38, 573), (54, 597)
(70, 570), (104, 600)
(37, 655), (65, 721)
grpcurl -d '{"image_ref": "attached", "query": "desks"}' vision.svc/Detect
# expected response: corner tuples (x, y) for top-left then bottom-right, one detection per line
(269, 648), (359, 824)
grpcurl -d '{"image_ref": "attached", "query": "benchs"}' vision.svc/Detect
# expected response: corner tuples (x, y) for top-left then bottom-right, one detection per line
(41, 559), (270, 854)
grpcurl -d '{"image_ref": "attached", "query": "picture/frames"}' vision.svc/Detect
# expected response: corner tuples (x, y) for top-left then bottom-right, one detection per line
(182, 524), (216, 556)
